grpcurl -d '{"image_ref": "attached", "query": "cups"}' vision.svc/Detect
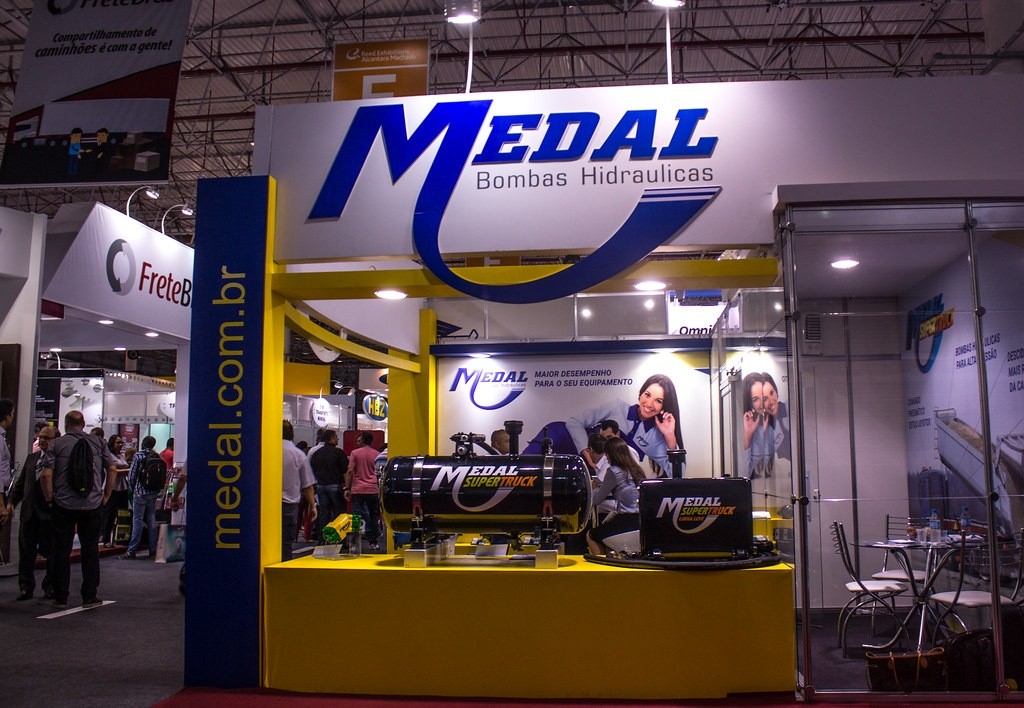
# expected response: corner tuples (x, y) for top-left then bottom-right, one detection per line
(917, 528), (927, 544)
(941, 530), (947, 544)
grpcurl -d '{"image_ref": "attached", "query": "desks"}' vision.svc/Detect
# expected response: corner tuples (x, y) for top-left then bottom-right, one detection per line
(850, 541), (991, 650)
(265, 553), (799, 701)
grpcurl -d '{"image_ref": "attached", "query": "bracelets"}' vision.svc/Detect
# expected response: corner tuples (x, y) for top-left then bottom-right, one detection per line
(345, 487), (350, 490)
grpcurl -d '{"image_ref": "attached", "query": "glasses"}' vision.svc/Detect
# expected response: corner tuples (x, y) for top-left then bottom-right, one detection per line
(38, 435), (57, 442)
(586, 448), (591, 452)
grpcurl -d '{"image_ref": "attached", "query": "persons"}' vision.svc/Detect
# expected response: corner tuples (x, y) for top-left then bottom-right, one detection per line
(488, 429), (510, 544)
(170, 463), (187, 593)
(118, 436), (159, 560)
(160, 438), (174, 484)
(90, 428), (130, 549)
(296, 430), (388, 543)
(0, 398), (61, 599)
(521, 374), (686, 478)
(282, 420), (317, 561)
(587, 419), (645, 554)
(41, 410), (118, 608)
(744, 372), (790, 479)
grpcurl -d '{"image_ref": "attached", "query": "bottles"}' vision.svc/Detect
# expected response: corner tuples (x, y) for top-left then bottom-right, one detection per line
(930, 509), (941, 545)
(961, 506), (972, 536)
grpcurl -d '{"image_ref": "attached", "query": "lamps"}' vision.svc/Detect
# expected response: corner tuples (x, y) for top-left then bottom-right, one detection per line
(647, 0), (687, 84)
(40, 352), (60, 369)
(161, 203), (193, 235)
(319, 380), (343, 398)
(442, 0), (482, 94)
(125, 185), (161, 217)
(336, 386), (356, 397)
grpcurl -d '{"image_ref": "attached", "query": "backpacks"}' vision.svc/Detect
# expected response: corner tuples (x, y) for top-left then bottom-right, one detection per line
(137, 452), (168, 492)
(64, 433), (94, 500)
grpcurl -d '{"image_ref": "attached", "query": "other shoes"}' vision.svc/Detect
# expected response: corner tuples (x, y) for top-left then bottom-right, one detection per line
(18, 587), (34, 601)
(148, 551), (155, 557)
(43, 587), (56, 600)
(117, 551), (136, 560)
(52, 597), (69, 608)
(83, 596), (104, 607)
(104, 541), (112, 548)
(370, 543), (380, 551)
(305, 538), (314, 543)
(361, 535), (368, 542)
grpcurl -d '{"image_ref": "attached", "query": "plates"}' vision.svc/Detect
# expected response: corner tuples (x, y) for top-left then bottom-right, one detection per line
(889, 540), (915, 544)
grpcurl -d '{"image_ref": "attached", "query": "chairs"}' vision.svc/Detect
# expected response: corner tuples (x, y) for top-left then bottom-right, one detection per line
(829, 520), (912, 658)
(870, 513), (944, 637)
(930, 528), (1024, 649)
